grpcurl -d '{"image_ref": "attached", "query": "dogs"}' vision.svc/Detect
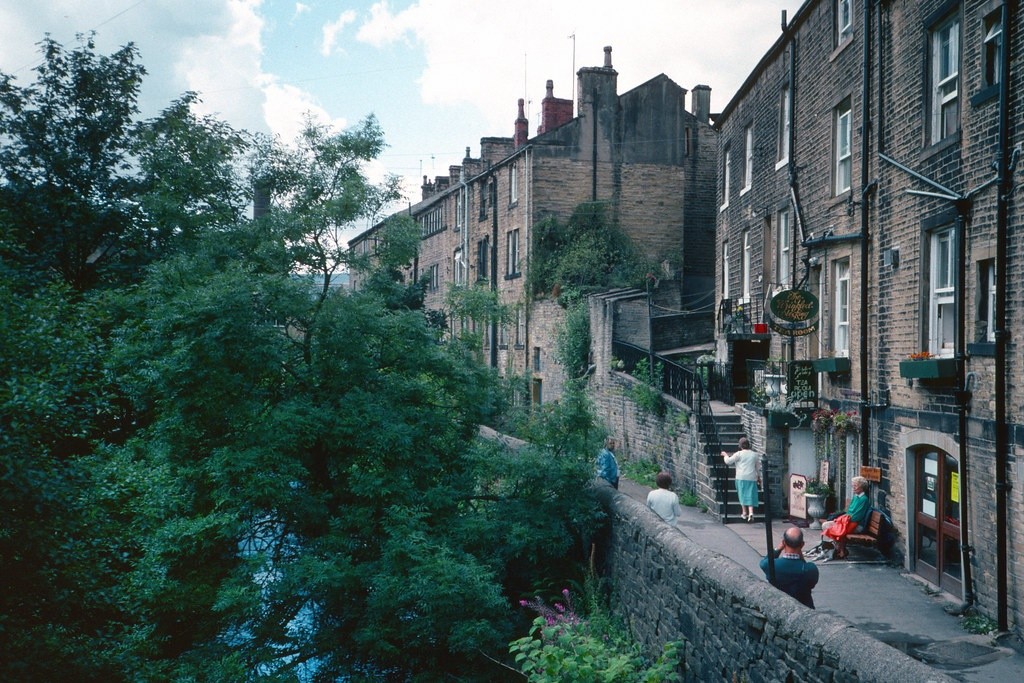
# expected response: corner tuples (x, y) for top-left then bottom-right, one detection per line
(803, 541), (836, 563)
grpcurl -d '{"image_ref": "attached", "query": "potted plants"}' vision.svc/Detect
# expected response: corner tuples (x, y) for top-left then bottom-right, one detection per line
(760, 364), (786, 409)
(796, 477), (836, 530)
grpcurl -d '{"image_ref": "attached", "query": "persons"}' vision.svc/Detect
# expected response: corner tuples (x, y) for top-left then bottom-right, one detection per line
(647, 471), (681, 527)
(822, 476), (869, 559)
(594, 436), (619, 489)
(721, 437), (762, 522)
(760, 527), (818, 609)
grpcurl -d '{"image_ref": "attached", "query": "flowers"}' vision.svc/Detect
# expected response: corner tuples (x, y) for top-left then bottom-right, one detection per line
(809, 407), (838, 449)
(907, 352), (936, 361)
(832, 410), (858, 439)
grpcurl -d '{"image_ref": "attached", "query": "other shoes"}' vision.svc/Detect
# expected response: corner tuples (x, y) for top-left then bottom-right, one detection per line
(741, 513), (747, 520)
(833, 547), (850, 560)
(746, 514), (754, 524)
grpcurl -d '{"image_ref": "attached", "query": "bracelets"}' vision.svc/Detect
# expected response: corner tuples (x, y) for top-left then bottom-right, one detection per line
(774, 548), (780, 555)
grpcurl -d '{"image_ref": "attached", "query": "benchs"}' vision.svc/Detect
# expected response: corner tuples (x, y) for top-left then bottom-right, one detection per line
(846, 510), (885, 561)
